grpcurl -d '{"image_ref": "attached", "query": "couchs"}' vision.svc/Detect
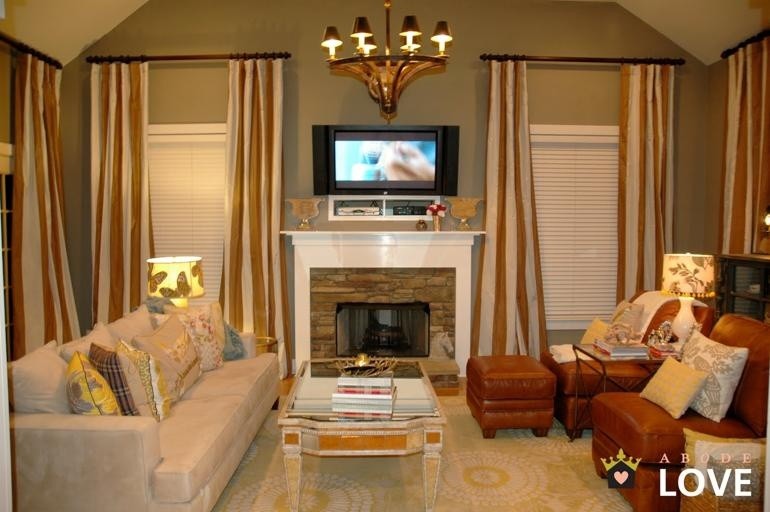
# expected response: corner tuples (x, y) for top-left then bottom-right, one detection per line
(8, 331), (280, 512)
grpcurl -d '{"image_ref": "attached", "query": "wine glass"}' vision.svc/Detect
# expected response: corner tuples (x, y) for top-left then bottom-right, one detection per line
(443, 196), (483, 232)
(285, 196), (327, 231)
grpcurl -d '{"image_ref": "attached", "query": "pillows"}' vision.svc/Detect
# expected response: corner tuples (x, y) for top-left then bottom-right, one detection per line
(8, 298), (244, 424)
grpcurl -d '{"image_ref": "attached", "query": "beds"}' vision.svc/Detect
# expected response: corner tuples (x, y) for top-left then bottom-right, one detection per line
(276, 357), (450, 512)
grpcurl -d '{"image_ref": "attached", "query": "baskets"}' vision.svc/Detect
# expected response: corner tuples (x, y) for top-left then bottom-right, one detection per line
(336, 357), (398, 378)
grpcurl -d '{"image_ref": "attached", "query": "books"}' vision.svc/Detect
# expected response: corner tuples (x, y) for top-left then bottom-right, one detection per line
(333, 389), (398, 405)
(332, 403), (395, 422)
(591, 336), (680, 360)
(337, 376), (393, 394)
(332, 386), (396, 399)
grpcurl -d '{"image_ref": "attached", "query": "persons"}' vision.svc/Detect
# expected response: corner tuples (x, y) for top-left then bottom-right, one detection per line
(376, 141), (435, 180)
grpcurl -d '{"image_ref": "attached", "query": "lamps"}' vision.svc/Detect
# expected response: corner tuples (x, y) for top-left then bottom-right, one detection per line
(660, 252), (717, 343)
(145, 256), (207, 307)
(320, 1), (455, 122)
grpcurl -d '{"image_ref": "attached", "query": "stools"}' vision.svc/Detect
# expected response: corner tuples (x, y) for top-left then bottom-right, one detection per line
(467, 354), (558, 439)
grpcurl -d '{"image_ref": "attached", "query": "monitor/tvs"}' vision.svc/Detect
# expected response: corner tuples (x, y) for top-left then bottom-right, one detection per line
(312, 125), (460, 195)
